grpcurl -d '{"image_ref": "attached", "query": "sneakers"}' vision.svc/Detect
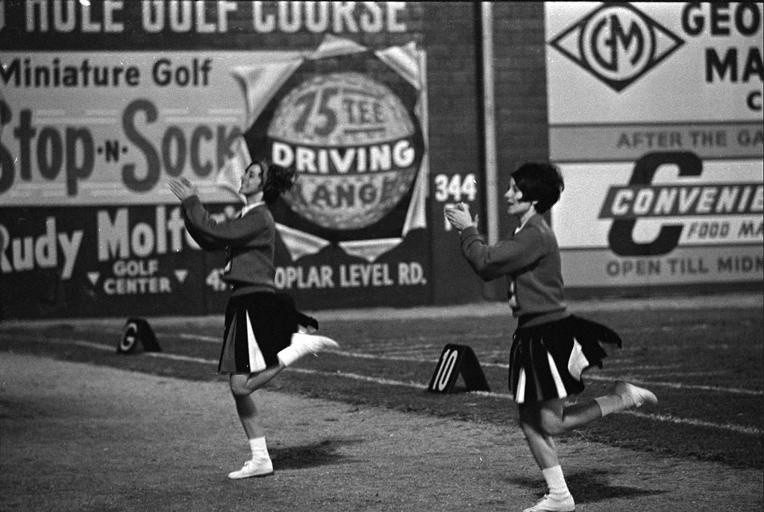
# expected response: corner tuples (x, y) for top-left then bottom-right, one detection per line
(522, 493), (575, 512)
(228, 458), (273, 480)
(612, 381), (660, 409)
(291, 332), (340, 357)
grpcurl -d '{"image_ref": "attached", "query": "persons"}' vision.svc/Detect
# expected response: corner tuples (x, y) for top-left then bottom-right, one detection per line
(444, 161), (659, 511)
(168, 159), (340, 481)
